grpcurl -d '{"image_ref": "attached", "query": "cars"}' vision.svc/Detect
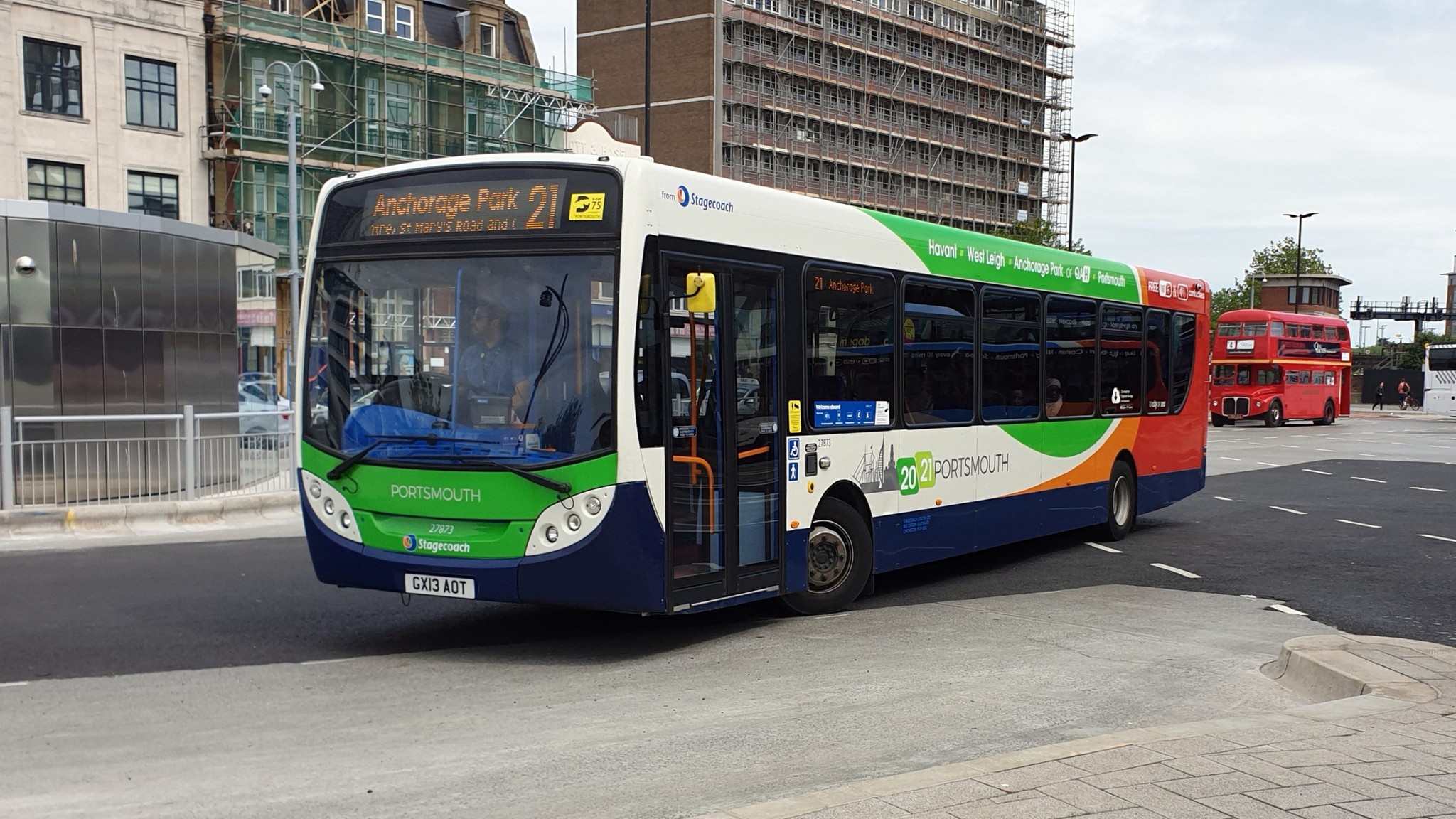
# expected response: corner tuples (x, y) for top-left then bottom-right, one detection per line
(236, 373), (295, 450)
(598, 368), (761, 417)
(310, 378), (381, 423)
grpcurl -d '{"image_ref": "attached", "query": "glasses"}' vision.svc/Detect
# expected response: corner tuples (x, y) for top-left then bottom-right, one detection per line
(472, 313), (495, 323)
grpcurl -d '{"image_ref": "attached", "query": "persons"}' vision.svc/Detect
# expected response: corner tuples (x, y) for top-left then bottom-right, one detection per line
(980, 313), (1063, 417)
(1373, 383), (1384, 410)
(456, 302), (530, 413)
(1398, 377), (1410, 405)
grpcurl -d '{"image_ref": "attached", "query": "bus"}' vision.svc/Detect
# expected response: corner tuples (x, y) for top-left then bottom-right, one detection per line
(805, 339), (1169, 422)
(294, 151), (1210, 617)
(1208, 309), (1353, 428)
(1422, 341), (1456, 413)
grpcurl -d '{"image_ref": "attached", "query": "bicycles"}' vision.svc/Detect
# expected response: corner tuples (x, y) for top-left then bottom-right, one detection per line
(1398, 392), (1420, 411)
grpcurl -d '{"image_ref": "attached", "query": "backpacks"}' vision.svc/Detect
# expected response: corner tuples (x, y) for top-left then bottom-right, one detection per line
(1400, 382), (1409, 393)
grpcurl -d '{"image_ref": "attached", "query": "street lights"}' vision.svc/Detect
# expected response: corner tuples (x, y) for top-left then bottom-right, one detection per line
(1282, 211), (1320, 338)
(257, 59), (324, 366)
(1248, 270), (1268, 335)
(512, 273), (572, 458)
(1361, 325), (1371, 353)
(1057, 132), (1098, 253)
